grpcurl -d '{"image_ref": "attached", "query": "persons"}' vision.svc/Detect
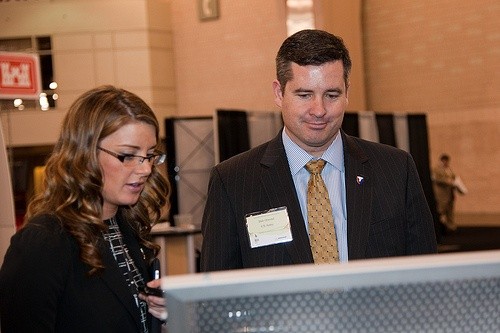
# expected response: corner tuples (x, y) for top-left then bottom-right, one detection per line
(0, 85), (169, 333)
(199, 29), (437, 272)
(437, 155), (455, 223)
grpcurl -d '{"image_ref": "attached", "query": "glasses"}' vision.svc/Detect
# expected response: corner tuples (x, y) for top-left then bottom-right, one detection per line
(96, 145), (166, 166)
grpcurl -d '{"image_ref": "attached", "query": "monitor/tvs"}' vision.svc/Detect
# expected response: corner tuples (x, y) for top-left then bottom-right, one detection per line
(160, 249), (500, 333)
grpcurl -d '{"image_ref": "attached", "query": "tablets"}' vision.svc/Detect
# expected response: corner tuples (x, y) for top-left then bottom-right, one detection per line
(151, 258), (160, 333)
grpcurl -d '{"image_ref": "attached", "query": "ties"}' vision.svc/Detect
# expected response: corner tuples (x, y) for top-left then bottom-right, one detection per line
(304, 159), (340, 264)
